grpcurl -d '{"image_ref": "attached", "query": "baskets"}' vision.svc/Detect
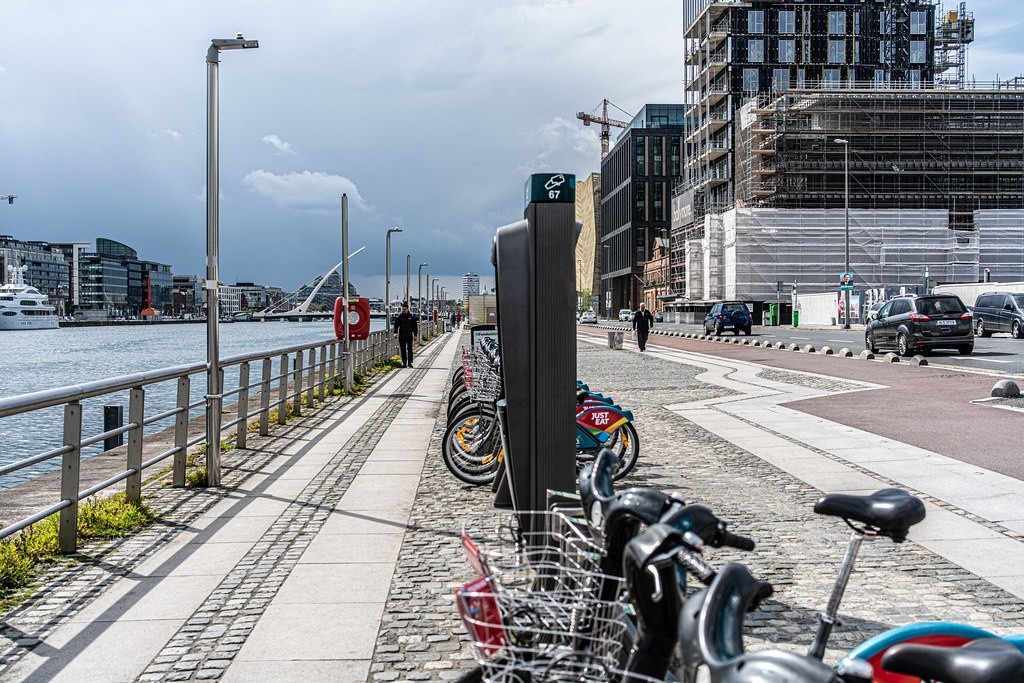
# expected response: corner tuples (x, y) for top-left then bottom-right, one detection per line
(461, 342), (501, 402)
(451, 510), (640, 683)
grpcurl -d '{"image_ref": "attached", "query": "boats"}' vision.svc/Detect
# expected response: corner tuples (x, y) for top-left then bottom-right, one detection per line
(0, 283), (61, 331)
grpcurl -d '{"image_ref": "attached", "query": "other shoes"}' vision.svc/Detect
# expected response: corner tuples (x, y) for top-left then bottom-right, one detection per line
(402, 365), (406, 368)
(408, 363), (413, 368)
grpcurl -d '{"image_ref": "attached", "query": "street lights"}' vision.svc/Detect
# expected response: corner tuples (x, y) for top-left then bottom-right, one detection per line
(441, 286), (450, 332)
(577, 260), (582, 316)
(205, 30), (260, 486)
(603, 245), (610, 321)
(419, 262), (428, 344)
(834, 138), (850, 329)
(385, 227), (404, 363)
(432, 277), (439, 336)
(661, 228), (667, 296)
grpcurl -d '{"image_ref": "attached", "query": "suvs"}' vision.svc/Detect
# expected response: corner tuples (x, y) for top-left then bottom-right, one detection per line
(703, 300), (754, 336)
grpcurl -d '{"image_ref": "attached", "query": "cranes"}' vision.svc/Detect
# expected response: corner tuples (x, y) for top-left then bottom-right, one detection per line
(576, 97), (635, 160)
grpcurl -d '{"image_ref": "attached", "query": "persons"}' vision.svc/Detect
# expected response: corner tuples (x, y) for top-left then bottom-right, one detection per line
(841, 274), (853, 286)
(451, 311), (465, 329)
(394, 304), (418, 368)
(633, 303), (653, 352)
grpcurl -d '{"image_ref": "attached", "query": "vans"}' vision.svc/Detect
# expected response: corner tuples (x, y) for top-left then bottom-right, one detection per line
(973, 289), (1024, 339)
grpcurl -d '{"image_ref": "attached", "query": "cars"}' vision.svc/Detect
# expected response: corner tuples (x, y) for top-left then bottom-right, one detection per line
(575, 311), (581, 321)
(863, 292), (974, 356)
(618, 309), (632, 322)
(580, 311), (597, 324)
(964, 305), (977, 334)
(628, 312), (637, 322)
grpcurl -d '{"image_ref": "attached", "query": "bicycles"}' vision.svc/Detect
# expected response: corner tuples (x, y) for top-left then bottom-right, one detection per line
(442, 335), (640, 482)
(454, 451), (1024, 683)
